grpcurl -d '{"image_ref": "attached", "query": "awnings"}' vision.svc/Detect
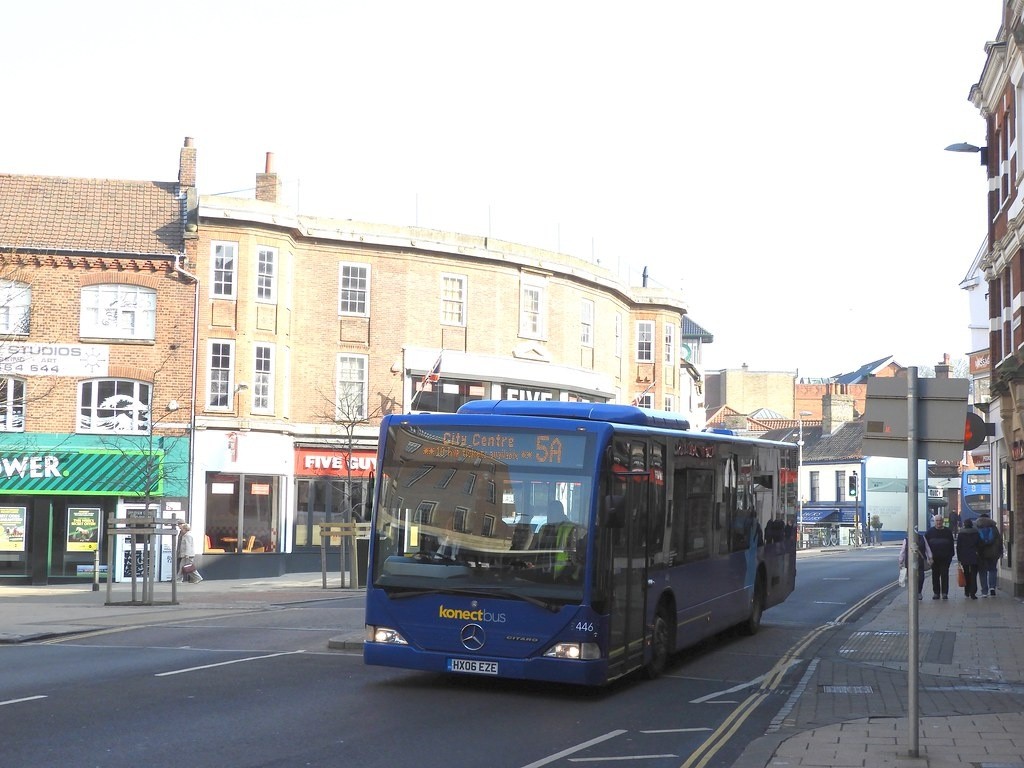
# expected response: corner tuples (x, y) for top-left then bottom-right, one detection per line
(928, 499), (948, 507)
(796, 510), (836, 523)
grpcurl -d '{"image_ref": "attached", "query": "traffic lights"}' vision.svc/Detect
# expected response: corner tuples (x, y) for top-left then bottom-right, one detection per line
(849, 475), (858, 497)
(962, 411), (987, 451)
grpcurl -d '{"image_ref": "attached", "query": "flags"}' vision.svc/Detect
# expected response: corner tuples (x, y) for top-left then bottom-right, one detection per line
(422, 356), (442, 384)
(632, 391), (647, 406)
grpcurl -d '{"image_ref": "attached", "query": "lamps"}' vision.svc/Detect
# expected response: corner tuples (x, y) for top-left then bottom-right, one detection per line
(943, 142), (988, 166)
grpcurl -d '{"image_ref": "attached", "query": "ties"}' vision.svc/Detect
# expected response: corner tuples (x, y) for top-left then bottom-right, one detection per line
(546, 526), (556, 570)
(447, 539), (453, 558)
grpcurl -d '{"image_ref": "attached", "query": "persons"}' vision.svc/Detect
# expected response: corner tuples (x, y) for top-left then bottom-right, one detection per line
(949, 509), (957, 538)
(177, 519), (203, 584)
(899, 528), (934, 600)
(975, 514), (1003, 597)
(417, 517), (467, 562)
(925, 515), (954, 599)
(957, 519), (992, 599)
(753, 512), (795, 547)
(523, 500), (576, 585)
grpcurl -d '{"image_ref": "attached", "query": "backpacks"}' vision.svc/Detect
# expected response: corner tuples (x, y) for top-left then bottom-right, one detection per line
(976, 526), (994, 544)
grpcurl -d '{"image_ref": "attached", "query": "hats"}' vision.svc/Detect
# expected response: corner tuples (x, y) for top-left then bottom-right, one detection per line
(177, 520), (185, 525)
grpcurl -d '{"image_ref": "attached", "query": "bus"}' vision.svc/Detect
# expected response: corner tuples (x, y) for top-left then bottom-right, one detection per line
(960, 469), (993, 528)
(362, 399), (801, 688)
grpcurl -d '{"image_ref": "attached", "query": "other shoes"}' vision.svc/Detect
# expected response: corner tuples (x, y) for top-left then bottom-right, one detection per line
(983, 594), (988, 598)
(193, 577), (203, 583)
(970, 594), (978, 599)
(965, 587), (969, 597)
(933, 593), (939, 599)
(942, 593), (948, 600)
(918, 593), (923, 600)
(991, 590), (996, 596)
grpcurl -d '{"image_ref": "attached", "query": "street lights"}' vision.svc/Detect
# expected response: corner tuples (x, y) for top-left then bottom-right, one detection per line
(798, 411), (813, 532)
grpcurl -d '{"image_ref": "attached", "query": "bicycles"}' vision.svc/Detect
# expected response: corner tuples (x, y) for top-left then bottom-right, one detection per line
(850, 526), (867, 546)
(821, 529), (838, 547)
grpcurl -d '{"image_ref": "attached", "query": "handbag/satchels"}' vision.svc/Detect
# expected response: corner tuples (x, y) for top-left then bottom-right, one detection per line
(183, 557), (195, 573)
(899, 566), (907, 587)
(958, 565), (966, 587)
(924, 559), (931, 571)
